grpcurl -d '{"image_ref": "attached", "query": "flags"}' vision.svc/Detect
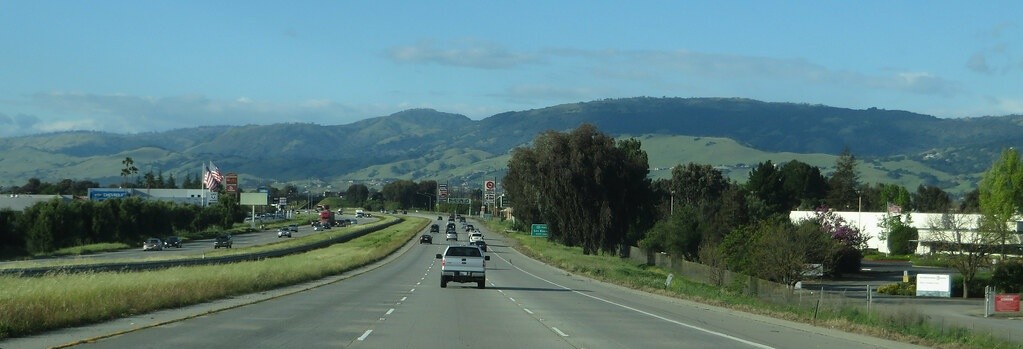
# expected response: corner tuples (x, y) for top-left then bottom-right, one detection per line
(888, 203), (902, 212)
(203, 161), (222, 192)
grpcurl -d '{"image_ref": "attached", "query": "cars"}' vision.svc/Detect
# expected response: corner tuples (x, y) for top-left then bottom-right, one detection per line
(470, 233), (485, 245)
(337, 209), (372, 218)
(435, 244), (491, 288)
(459, 217), (466, 223)
(278, 227), (291, 238)
(288, 224), (298, 232)
(468, 229), (481, 238)
(445, 223), (458, 241)
(213, 234), (234, 249)
(448, 216), (455, 222)
(466, 224), (474, 232)
(419, 234), (433, 245)
(311, 219), (358, 232)
(456, 214), (461, 219)
(164, 236), (182, 248)
(143, 238), (162, 251)
(476, 240), (488, 252)
(437, 216), (442, 220)
(430, 224), (439, 233)
